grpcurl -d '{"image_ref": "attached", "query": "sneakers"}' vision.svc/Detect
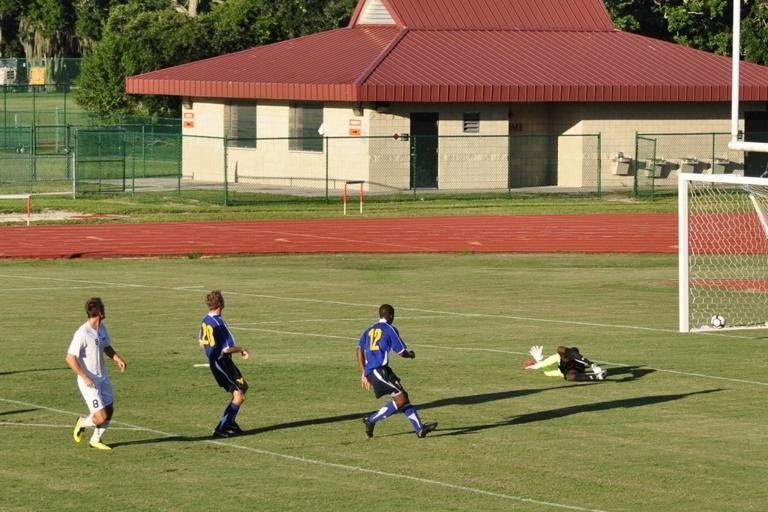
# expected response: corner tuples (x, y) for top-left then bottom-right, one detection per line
(213, 422), (245, 437)
(74, 417), (85, 442)
(590, 362), (608, 381)
(90, 440), (112, 450)
(418, 422), (437, 438)
(363, 414), (374, 437)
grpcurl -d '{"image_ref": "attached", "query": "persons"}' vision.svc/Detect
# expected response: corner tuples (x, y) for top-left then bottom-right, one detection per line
(525, 345), (606, 381)
(198, 291), (248, 437)
(66, 297), (127, 452)
(356, 304), (437, 439)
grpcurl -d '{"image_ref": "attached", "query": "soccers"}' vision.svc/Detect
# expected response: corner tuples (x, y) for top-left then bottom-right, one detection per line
(710, 315), (725, 328)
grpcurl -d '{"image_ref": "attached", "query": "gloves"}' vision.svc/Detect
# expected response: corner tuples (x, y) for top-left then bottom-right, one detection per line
(529, 345), (544, 362)
